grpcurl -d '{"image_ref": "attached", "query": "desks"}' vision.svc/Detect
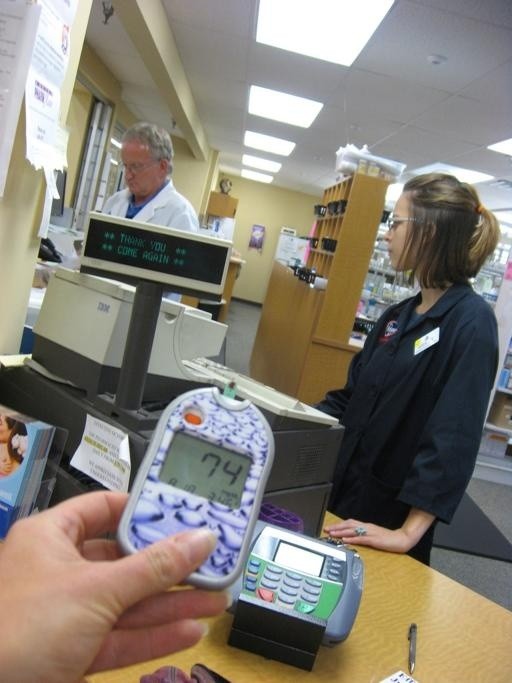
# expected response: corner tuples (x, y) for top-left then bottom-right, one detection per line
(0, 354), (512, 683)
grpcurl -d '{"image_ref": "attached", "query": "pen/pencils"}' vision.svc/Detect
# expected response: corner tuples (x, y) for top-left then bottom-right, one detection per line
(408, 623), (417, 674)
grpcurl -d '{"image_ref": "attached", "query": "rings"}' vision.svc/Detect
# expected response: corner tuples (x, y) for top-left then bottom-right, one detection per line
(356, 526), (367, 537)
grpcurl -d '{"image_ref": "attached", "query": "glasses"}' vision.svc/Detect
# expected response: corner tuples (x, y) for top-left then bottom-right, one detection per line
(387, 217), (426, 230)
(119, 162), (158, 173)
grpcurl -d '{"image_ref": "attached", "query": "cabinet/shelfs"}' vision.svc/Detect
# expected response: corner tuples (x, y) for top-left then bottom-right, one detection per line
(182, 191), (242, 322)
(473, 254), (512, 487)
(249, 173), (391, 406)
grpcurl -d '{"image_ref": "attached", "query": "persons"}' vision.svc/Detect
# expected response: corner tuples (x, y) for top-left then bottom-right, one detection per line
(0, 490), (233, 683)
(312, 172), (499, 566)
(1, 416), (28, 479)
(101, 122), (199, 305)
